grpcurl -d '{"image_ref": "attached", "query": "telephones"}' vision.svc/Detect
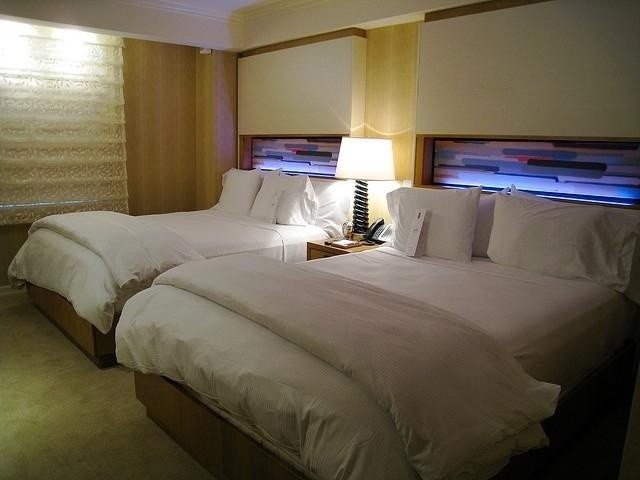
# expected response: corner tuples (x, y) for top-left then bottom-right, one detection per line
(367, 218), (394, 243)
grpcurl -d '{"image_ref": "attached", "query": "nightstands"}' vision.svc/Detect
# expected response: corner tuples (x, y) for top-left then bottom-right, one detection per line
(306, 236), (382, 261)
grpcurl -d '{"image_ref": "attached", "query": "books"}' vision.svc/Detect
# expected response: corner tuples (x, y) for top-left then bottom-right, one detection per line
(404, 208), (429, 257)
(331, 239), (362, 249)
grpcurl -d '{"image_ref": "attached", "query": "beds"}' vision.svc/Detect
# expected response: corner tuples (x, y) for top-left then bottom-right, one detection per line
(7, 176), (349, 370)
(116, 244), (639, 478)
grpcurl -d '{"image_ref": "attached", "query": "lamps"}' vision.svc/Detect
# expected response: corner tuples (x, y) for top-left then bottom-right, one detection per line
(335, 137), (397, 241)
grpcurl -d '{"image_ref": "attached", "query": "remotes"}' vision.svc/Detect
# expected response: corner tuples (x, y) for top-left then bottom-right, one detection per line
(325, 239), (334, 245)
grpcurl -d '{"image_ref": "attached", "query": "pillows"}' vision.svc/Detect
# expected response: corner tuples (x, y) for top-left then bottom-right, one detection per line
(510, 183), (544, 200)
(473, 186), (509, 258)
(385, 184), (483, 264)
(219, 168), (316, 228)
(488, 192), (640, 293)
